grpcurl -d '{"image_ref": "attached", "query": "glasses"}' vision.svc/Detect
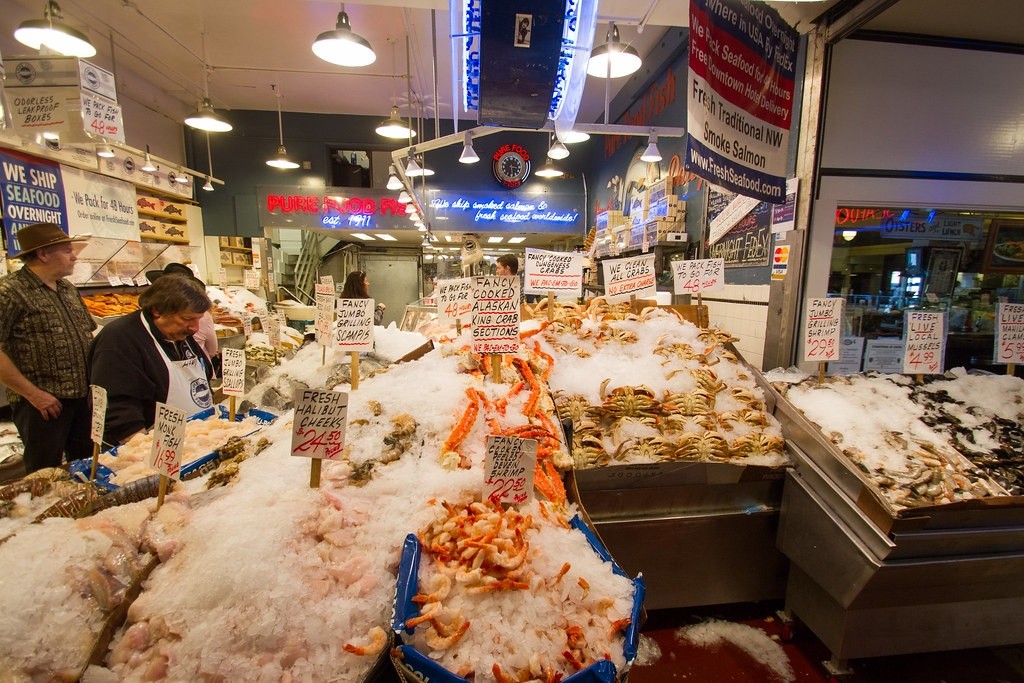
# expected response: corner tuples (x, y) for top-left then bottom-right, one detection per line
(360, 270), (364, 277)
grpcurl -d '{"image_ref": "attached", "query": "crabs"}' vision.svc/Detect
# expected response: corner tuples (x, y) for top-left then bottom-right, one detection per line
(433, 282), (782, 529)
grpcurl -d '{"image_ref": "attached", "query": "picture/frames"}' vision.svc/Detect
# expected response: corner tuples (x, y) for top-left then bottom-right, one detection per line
(981, 219), (1024, 274)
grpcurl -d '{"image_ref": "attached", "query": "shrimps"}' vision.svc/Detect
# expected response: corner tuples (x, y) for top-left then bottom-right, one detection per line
(0, 347), (420, 612)
(773, 375), (991, 517)
(344, 494), (633, 683)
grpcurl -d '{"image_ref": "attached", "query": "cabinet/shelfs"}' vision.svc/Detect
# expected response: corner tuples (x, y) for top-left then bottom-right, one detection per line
(219, 245), (253, 267)
(582, 241), (693, 303)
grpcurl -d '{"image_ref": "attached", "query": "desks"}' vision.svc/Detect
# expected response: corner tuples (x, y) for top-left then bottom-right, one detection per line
(944, 332), (1009, 375)
(271, 305), (316, 321)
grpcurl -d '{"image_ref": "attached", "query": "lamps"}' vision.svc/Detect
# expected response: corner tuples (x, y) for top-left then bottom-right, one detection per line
(535, 128), (590, 178)
(641, 130), (663, 161)
(265, 88), (304, 170)
(843, 231), (857, 241)
(587, 22), (643, 79)
(374, 38), (417, 137)
(174, 170), (188, 182)
(179, 33), (234, 132)
(387, 148), (435, 250)
(310, 4), (377, 67)
(13, 0), (97, 58)
(141, 153), (156, 171)
(97, 145), (115, 158)
(459, 132), (481, 163)
(202, 176), (214, 191)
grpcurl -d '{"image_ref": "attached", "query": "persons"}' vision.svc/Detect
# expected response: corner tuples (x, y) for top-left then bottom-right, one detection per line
(340, 269), (386, 326)
(0, 223), (98, 475)
(496, 253), (524, 305)
(146, 263), (218, 379)
(65, 274), (212, 454)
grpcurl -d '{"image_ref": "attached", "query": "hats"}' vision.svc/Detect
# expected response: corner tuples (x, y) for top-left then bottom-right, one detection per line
(12, 222), (87, 258)
(146, 263), (206, 290)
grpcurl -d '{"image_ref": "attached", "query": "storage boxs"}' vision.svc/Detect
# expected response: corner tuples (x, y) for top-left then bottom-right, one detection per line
(219, 236), (248, 264)
(593, 177), (687, 253)
(0, 58), (125, 140)
